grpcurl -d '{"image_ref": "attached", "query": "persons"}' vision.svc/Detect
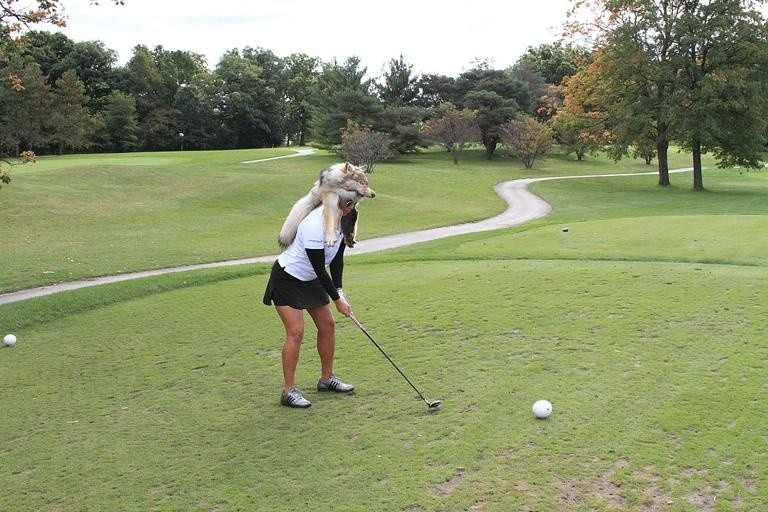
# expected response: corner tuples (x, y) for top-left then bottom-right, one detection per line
(260, 188), (363, 410)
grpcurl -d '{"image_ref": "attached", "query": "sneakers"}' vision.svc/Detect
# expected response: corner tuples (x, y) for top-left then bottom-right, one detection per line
(281, 387), (311, 408)
(317, 375), (354, 392)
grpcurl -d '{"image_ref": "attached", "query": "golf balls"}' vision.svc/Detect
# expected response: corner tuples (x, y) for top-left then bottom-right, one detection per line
(3, 333), (16, 346)
(532, 399), (552, 417)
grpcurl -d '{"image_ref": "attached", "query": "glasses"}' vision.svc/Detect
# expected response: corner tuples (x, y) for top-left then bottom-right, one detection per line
(343, 200), (357, 207)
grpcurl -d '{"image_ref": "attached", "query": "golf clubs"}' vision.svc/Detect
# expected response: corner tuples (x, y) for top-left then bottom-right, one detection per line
(347, 314), (440, 407)
